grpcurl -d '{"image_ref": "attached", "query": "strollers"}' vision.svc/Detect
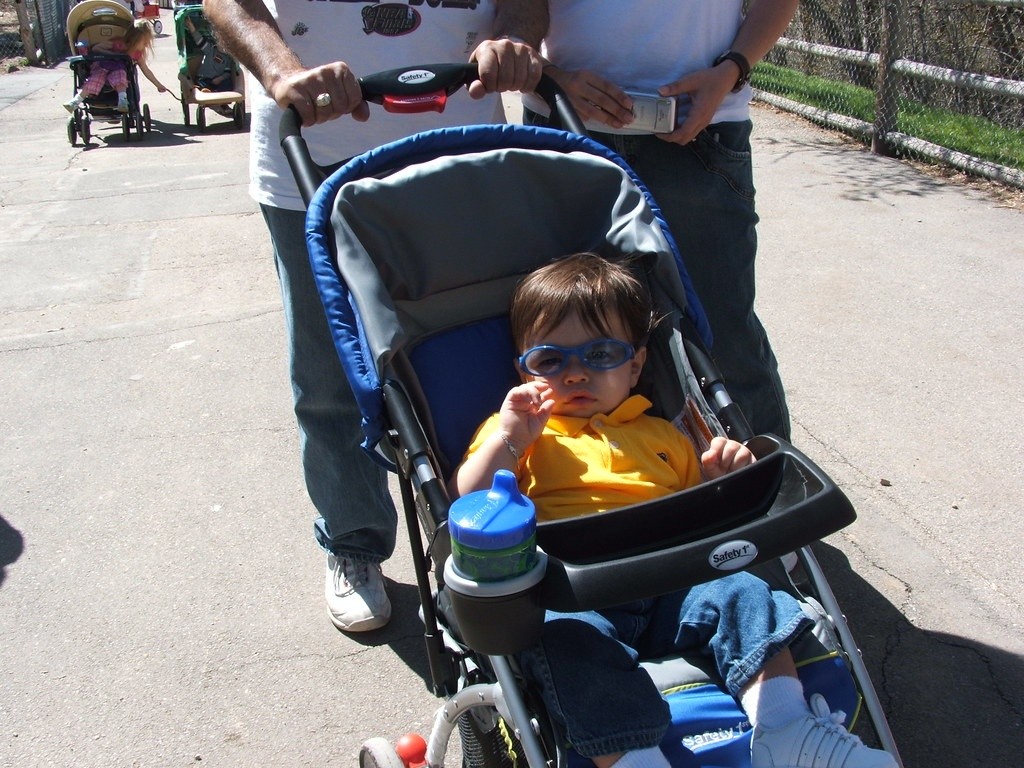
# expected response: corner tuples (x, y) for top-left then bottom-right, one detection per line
(276, 58), (907, 768)
(61, 0), (154, 146)
(174, 0), (249, 131)
(131, 0), (163, 34)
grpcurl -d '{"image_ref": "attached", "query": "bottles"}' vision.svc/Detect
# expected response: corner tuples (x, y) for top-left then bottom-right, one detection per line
(448, 469), (538, 583)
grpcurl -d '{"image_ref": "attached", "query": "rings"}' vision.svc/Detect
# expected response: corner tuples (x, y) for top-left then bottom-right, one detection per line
(313, 93), (331, 108)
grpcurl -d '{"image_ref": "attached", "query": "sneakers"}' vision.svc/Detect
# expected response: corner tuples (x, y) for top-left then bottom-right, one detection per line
(748, 693), (898, 768)
(324, 547), (392, 634)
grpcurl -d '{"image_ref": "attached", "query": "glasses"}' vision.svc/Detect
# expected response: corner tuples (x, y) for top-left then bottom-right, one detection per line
(518, 338), (636, 376)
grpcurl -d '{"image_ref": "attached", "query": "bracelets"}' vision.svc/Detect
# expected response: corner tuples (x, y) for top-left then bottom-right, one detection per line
(495, 427), (521, 472)
(712, 49), (752, 94)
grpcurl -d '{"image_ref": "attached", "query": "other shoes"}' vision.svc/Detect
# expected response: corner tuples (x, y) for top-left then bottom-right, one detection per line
(63, 101), (78, 114)
(195, 78), (213, 92)
(118, 100), (130, 113)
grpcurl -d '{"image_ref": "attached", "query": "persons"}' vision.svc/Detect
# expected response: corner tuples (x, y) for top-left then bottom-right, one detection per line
(445, 250), (901, 768)
(545, 1), (803, 577)
(63, 18), (167, 113)
(203, 0), (550, 633)
(185, 14), (237, 94)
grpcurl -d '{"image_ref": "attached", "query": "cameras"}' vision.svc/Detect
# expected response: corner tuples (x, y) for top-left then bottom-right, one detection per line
(621, 91), (675, 134)
